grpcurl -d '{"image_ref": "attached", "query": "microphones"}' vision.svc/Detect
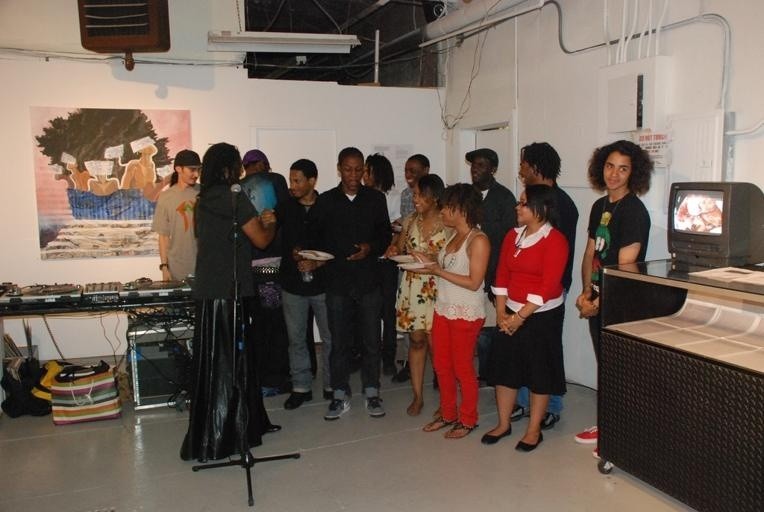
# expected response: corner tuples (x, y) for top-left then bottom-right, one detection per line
(230, 185), (244, 229)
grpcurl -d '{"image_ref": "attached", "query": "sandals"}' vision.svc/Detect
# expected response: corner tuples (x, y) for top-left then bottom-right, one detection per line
(407, 397), (479, 440)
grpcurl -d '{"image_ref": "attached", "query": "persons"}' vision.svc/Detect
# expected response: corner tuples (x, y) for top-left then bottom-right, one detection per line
(575, 140), (654, 458)
(180, 143), (275, 462)
(151, 151), (203, 282)
(385, 141), (580, 451)
(242, 147), (403, 422)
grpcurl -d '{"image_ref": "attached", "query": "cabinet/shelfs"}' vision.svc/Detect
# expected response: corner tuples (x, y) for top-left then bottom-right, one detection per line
(596, 259), (764, 512)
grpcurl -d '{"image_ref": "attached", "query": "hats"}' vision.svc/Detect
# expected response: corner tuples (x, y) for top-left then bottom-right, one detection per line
(242, 149), (267, 167)
(174, 149), (202, 171)
(465, 148), (500, 168)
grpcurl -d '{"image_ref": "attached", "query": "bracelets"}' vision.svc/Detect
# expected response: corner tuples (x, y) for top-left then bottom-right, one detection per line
(590, 302), (600, 311)
(160, 263), (169, 271)
(583, 285), (591, 289)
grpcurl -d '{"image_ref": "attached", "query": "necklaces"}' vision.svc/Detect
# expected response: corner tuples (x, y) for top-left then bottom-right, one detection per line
(602, 195), (619, 223)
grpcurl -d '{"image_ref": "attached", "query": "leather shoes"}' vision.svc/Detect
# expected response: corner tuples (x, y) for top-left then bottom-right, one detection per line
(516, 430), (543, 452)
(283, 389), (313, 410)
(323, 386), (334, 401)
(481, 423), (512, 444)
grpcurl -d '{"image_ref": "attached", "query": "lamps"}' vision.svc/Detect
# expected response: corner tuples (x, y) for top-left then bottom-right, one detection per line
(206, 0), (362, 53)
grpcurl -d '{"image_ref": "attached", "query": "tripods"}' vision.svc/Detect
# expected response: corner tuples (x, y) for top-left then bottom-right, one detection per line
(192, 245), (300, 506)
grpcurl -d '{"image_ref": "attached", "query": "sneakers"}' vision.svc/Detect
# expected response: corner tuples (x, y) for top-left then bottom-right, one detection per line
(363, 395), (386, 417)
(348, 358), (440, 390)
(510, 404), (561, 429)
(575, 426), (599, 460)
(325, 399), (351, 420)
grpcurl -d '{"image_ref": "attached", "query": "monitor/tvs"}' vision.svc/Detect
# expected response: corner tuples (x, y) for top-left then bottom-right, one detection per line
(668, 181), (764, 268)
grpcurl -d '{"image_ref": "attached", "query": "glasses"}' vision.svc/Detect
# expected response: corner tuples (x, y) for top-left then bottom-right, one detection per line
(515, 200), (528, 207)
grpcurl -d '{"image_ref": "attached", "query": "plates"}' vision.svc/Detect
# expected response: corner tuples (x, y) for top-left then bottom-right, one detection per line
(299, 250), (336, 262)
(393, 254), (437, 270)
